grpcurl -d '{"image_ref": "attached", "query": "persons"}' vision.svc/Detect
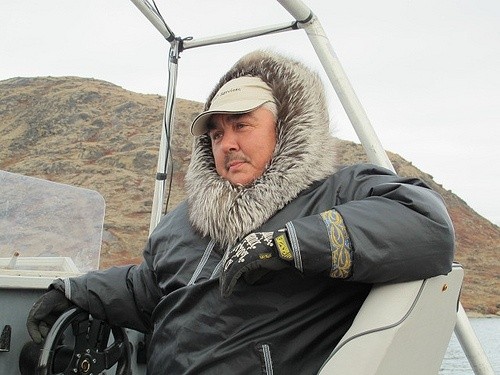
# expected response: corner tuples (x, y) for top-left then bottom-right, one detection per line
(26, 49), (455, 375)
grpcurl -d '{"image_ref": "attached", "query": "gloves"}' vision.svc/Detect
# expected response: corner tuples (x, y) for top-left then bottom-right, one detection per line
(219, 228), (294, 297)
(26, 278), (76, 345)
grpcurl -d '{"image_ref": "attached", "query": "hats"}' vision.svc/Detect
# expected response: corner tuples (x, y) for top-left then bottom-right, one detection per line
(190, 76), (277, 137)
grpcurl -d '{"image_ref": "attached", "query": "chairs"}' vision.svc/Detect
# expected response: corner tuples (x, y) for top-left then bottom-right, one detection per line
(316, 261), (464, 375)
